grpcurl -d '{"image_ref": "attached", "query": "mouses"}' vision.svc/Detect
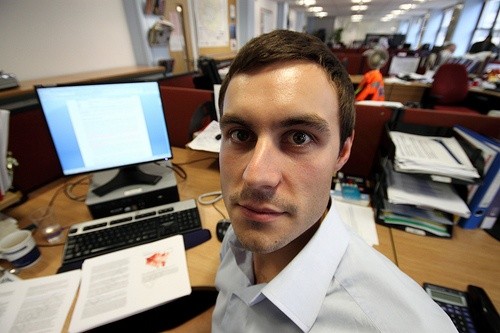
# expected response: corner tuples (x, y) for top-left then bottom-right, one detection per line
(216, 219), (231, 243)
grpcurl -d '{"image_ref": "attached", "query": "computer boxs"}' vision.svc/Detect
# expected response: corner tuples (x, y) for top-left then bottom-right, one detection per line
(85, 160), (180, 220)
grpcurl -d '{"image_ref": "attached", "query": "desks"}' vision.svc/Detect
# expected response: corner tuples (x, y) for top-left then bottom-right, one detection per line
(0, 45), (500, 333)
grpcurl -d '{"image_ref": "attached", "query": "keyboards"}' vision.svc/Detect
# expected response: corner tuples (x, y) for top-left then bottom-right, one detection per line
(61, 198), (201, 263)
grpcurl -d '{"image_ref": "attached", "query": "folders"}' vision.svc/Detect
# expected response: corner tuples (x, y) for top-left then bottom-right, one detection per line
(452, 125), (500, 230)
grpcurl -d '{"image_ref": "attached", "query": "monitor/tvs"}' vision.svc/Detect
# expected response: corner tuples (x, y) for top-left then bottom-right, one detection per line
(389, 56), (421, 77)
(34, 79), (174, 197)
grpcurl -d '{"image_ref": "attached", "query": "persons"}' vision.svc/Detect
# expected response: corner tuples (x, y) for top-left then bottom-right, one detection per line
(469, 33), (499, 60)
(429, 43), (456, 70)
(157, 29), (459, 333)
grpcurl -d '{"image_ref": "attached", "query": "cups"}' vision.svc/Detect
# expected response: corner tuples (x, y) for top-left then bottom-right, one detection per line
(29, 207), (64, 242)
(0, 230), (41, 273)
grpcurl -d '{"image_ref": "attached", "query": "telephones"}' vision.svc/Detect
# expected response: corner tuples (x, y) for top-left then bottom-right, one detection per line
(422, 281), (500, 333)
